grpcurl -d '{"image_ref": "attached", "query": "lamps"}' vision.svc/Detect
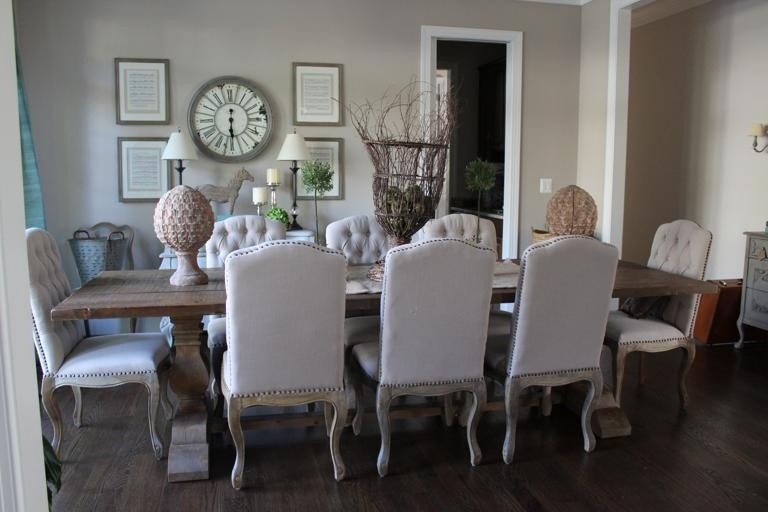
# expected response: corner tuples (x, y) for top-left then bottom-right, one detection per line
(277, 130), (310, 230)
(748, 122), (768, 154)
(162, 128), (198, 186)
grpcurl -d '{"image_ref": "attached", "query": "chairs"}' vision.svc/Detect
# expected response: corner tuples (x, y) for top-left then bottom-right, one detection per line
(353, 236), (497, 477)
(322, 213), (392, 263)
(22, 227), (173, 462)
(205, 209), (285, 267)
(605, 218), (714, 413)
(486, 235), (619, 466)
(221, 239), (348, 491)
(423, 212), (498, 257)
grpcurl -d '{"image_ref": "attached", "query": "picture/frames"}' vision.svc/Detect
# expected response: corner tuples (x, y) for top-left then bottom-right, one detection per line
(116, 135), (174, 204)
(297, 137), (346, 202)
(292, 62), (345, 127)
(113, 57), (172, 124)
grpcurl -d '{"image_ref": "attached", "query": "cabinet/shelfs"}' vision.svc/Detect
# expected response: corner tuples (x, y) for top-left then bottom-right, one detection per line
(734, 230), (768, 350)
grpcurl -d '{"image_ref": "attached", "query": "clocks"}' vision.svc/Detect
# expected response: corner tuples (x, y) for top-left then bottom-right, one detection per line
(187, 74), (276, 164)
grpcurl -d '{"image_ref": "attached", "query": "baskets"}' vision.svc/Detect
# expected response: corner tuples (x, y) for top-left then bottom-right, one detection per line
(67, 230), (128, 289)
(532, 228), (595, 245)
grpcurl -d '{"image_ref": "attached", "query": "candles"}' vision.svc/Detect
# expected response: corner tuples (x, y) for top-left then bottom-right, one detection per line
(266, 168), (282, 185)
(252, 187), (266, 203)
